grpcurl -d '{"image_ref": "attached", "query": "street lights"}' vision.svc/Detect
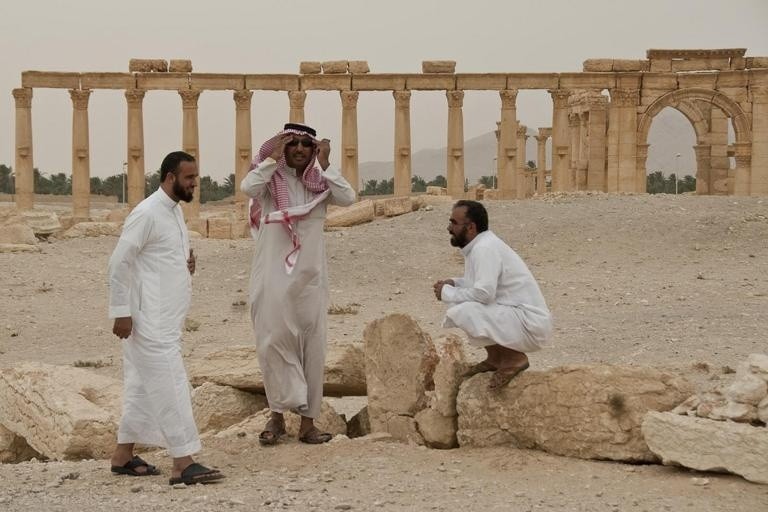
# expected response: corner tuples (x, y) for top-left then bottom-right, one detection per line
(675, 153), (681, 194)
(123, 163), (128, 204)
(493, 158), (497, 189)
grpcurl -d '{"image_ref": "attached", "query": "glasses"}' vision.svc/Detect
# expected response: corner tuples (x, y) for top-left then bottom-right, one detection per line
(288, 139), (312, 146)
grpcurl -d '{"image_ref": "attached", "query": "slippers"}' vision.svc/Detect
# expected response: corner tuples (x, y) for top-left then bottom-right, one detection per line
(464, 362), (529, 390)
(259, 419), (285, 443)
(169, 463), (224, 485)
(299, 426), (332, 444)
(111, 455), (160, 475)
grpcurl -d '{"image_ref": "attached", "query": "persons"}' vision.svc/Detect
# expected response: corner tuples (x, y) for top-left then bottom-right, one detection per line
(433, 199), (552, 391)
(240, 123), (356, 445)
(107, 151), (226, 485)
